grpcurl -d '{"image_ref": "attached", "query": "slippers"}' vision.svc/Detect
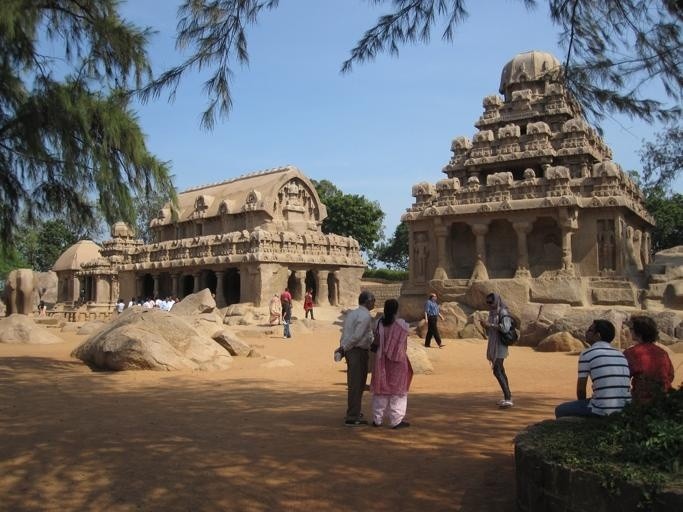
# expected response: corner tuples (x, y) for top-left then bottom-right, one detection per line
(496, 399), (506, 405)
(499, 401), (514, 408)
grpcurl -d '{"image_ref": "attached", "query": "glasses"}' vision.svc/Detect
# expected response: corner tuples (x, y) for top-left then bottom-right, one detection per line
(486, 301), (493, 304)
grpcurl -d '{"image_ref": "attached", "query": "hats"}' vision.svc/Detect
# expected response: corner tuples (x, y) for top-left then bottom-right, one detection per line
(622, 316), (656, 333)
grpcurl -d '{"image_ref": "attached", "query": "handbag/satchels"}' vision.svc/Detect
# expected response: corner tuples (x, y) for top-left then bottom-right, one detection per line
(370, 332), (380, 352)
(500, 326), (518, 346)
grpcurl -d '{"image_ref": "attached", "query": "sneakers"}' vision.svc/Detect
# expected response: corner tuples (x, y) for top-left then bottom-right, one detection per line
(345, 413), (410, 428)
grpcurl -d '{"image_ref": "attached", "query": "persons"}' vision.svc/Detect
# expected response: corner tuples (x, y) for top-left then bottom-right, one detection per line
(270, 288), (295, 339)
(303, 288), (317, 317)
(112, 296), (179, 317)
(37, 301), (48, 317)
(332, 290), (375, 426)
(475, 295), (521, 409)
(422, 292), (447, 348)
(81, 289), (86, 304)
(556, 324), (633, 419)
(621, 315), (675, 402)
(370, 300), (416, 428)
